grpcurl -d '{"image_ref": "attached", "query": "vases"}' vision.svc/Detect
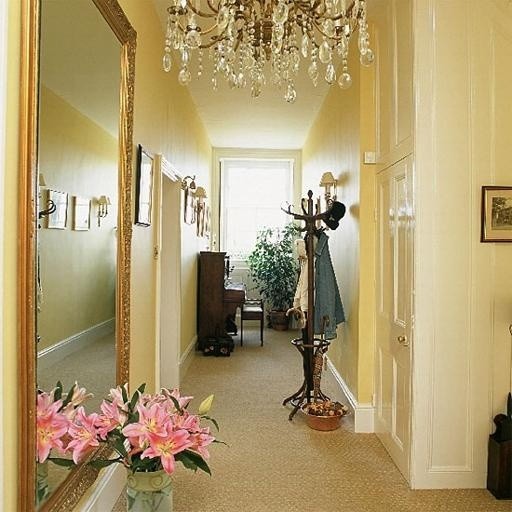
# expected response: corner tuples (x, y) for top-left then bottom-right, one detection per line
(126, 468), (173, 512)
(35, 462), (50, 506)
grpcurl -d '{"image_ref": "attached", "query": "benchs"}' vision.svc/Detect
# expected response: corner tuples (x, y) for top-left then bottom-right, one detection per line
(241, 299), (264, 347)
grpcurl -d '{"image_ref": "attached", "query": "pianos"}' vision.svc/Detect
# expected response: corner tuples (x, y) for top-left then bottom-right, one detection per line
(197, 251), (246, 351)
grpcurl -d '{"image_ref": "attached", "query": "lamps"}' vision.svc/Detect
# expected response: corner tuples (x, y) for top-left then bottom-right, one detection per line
(161, 0), (376, 104)
(319, 172), (338, 209)
(97, 196), (112, 226)
(192, 187), (207, 204)
(39, 174), (46, 186)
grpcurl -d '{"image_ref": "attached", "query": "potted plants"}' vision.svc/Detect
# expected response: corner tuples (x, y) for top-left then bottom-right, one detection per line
(245, 222), (301, 331)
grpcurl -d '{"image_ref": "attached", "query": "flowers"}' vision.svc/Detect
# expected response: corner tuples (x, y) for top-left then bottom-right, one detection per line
(64, 382), (229, 477)
(36, 380), (95, 464)
(303, 402), (348, 416)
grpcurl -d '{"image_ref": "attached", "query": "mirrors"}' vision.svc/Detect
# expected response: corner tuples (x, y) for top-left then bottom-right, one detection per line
(18, 0), (137, 511)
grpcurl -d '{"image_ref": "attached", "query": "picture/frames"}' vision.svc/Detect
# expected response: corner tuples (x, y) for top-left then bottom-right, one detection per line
(134, 144), (155, 227)
(480, 185), (512, 242)
(46, 189), (68, 230)
(72, 196), (92, 231)
